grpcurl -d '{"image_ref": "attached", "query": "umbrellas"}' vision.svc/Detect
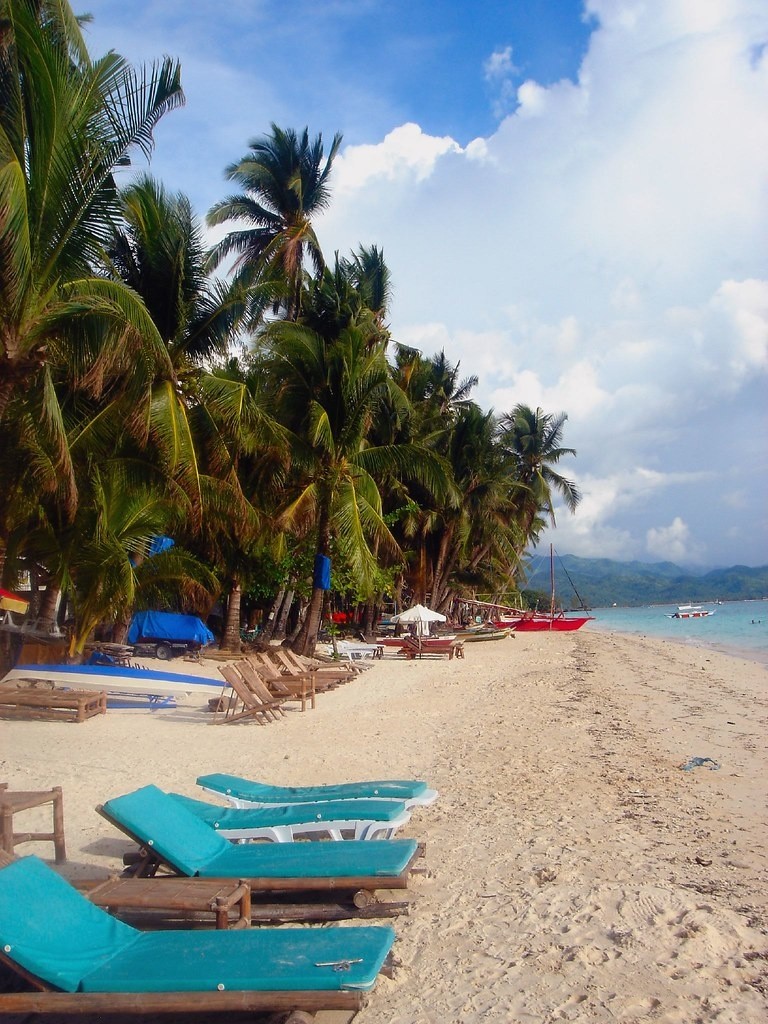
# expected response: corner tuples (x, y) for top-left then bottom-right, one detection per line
(391, 603), (446, 658)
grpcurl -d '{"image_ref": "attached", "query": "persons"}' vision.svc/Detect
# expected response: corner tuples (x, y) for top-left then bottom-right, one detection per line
(407, 624), (416, 636)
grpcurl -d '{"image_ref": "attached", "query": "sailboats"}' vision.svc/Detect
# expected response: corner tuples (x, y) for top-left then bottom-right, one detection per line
(478, 542), (597, 631)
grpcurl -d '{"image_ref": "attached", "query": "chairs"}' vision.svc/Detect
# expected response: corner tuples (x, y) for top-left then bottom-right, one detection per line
(0, 851), (397, 1024)
(94, 784), (426, 921)
(397, 634), (466, 660)
(196, 772), (438, 839)
(167, 792), (411, 842)
(206, 640), (386, 726)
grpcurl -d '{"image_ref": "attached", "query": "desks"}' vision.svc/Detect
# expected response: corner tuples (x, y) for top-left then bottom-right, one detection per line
(0, 781), (67, 863)
(0, 687), (106, 723)
(86, 874), (253, 929)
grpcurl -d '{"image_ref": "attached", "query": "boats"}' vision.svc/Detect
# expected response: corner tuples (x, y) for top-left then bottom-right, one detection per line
(664, 608), (718, 619)
(678, 600), (703, 610)
(436, 626), (517, 643)
